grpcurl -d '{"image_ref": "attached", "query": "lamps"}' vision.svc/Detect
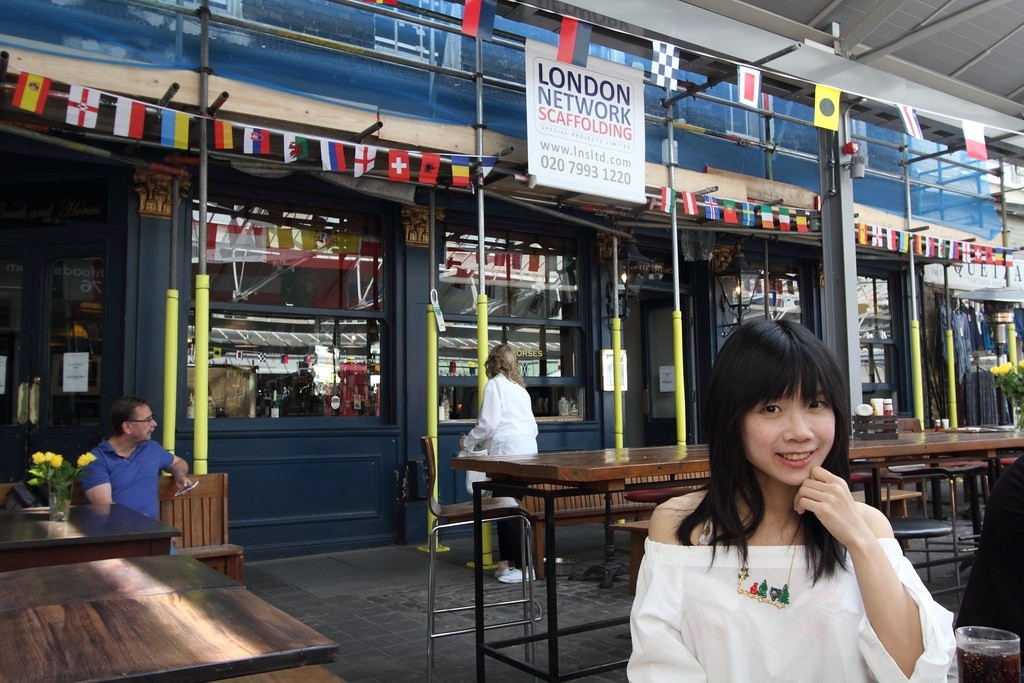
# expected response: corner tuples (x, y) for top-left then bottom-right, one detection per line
(842, 156), (865, 179)
(603, 229), (654, 334)
(716, 244), (761, 337)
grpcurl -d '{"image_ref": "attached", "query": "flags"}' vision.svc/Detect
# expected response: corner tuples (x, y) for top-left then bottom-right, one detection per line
(476, 246), (557, 272)
(737, 64), (762, 108)
(558, 17), (592, 68)
(857, 223), (1013, 268)
(12, 71), (498, 187)
(462, 0), (497, 44)
(652, 39), (679, 90)
(814, 85), (841, 131)
(962, 119), (988, 160)
(192, 220), (380, 257)
(363, 0), (398, 5)
(661, 187), (808, 232)
(893, 104), (924, 141)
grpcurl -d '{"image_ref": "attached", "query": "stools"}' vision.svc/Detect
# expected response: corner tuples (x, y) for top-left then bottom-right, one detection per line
(881, 418), (961, 604)
(420, 436), (543, 683)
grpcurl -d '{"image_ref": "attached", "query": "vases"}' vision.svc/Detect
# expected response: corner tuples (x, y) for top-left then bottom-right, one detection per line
(48, 482), (73, 521)
(1014, 396), (1024, 432)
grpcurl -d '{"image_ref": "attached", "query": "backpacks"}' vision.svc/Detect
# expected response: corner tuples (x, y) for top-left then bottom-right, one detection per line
(6, 480), (48, 511)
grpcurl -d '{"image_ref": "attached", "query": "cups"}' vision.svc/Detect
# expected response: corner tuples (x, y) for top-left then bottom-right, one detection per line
(955, 625), (1021, 683)
(942, 419), (949, 429)
(439, 406), (445, 421)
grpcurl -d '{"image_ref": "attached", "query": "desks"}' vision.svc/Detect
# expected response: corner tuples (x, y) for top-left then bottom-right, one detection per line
(0, 504), (181, 572)
(451, 426), (1024, 683)
(0, 555), (339, 683)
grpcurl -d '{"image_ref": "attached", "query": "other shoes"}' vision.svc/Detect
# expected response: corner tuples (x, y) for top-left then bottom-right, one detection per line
(496, 566), (536, 583)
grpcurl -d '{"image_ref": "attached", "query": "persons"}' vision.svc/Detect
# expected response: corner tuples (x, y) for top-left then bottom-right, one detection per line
(78, 397), (192, 556)
(460, 344), (537, 583)
(628, 317), (959, 683)
(955, 454), (1024, 683)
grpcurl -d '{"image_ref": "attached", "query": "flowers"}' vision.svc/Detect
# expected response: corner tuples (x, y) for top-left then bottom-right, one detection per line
(27, 451), (97, 516)
(990, 360), (1024, 429)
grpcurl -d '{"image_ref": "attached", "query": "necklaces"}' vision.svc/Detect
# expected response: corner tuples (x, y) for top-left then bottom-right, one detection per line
(736, 515), (801, 609)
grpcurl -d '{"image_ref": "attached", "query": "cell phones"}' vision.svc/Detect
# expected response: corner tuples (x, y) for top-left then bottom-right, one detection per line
(174, 481), (199, 496)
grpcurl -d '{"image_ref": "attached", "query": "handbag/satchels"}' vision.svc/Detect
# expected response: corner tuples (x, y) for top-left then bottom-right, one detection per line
(466, 449), (492, 496)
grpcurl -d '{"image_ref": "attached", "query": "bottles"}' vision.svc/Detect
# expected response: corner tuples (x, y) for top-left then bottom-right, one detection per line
(883, 398), (893, 433)
(441, 393), (449, 422)
(255, 385), (377, 417)
(531, 397), (579, 417)
(935, 418), (941, 432)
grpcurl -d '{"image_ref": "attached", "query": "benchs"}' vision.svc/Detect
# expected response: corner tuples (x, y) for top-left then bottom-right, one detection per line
(522, 457), (1018, 579)
(0, 473), (244, 586)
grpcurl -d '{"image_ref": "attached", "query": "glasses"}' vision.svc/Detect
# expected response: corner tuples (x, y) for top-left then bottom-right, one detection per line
(130, 416), (158, 423)
